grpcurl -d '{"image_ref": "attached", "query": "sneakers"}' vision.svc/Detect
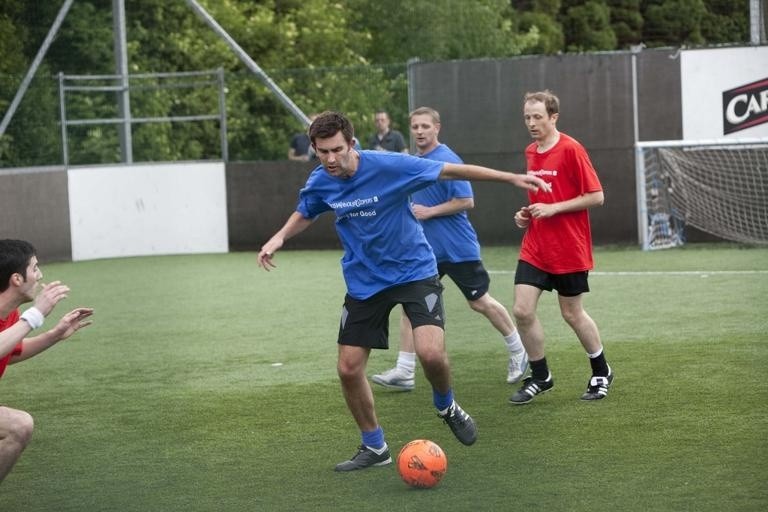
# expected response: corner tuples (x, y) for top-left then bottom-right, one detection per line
(371, 369), (415, 390)
(507, 369), (553, 404)
(580, 363), (614, 400)
(506, 351), (528, 384)
(438, 399), (477, 445)
(335, 441), (393, 471)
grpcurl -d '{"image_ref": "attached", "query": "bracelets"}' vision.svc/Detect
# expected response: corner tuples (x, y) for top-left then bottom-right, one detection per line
(19, 306), (45, 330)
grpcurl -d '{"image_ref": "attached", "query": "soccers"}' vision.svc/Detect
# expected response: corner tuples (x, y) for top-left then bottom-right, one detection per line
(398, 439), (447, 488)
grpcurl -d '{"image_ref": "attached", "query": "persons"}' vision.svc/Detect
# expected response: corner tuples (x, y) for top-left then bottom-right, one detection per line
(286, 115), (321, 160)
(1, 239), (94, 486)
(257, 111), (553, 472)
(371, 112), (408, 155)
(508, 90), (614, 405)
(370, 106), (530, 390)
